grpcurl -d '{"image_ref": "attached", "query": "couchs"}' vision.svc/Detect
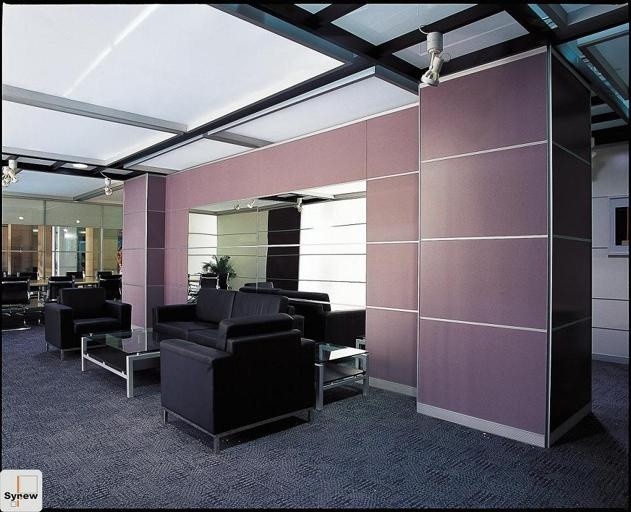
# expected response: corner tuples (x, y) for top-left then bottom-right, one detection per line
(44, 287), (132, 360)
(239, 287), (365, 350)
(159, 312), (319, 450)
(152, 288), (288, 350)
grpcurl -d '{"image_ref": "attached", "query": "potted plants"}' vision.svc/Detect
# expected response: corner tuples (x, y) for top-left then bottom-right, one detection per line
(201, 254), (236, 287)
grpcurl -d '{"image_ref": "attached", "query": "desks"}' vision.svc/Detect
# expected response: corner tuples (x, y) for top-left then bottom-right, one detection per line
(355, 334), (368, 372)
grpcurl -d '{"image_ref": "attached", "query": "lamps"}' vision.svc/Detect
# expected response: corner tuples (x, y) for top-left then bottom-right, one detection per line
(418, 24), (451, 87)
(232, 200), (240, 215)
(1, 159), (24, 187)
(296, 198), (303, 214)
(247, 199), (255, 209)
(104, 177), (113, 196)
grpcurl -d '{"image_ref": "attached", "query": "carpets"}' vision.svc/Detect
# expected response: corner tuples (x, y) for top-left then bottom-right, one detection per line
(0, 320), (631, 510)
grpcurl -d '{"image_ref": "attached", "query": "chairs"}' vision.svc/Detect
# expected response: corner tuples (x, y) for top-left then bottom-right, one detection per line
(98, 274), (122, 300)
(16, 272), (41, 325)
(33, 276), (75, 315)
(245, 283), (256, 287)
(257, 282), (274, 289)
(0, 276), (32, 332)
(64, 271), (84, 288)
(96, 270), (112, 281)
(199, 272), (218, 289)
